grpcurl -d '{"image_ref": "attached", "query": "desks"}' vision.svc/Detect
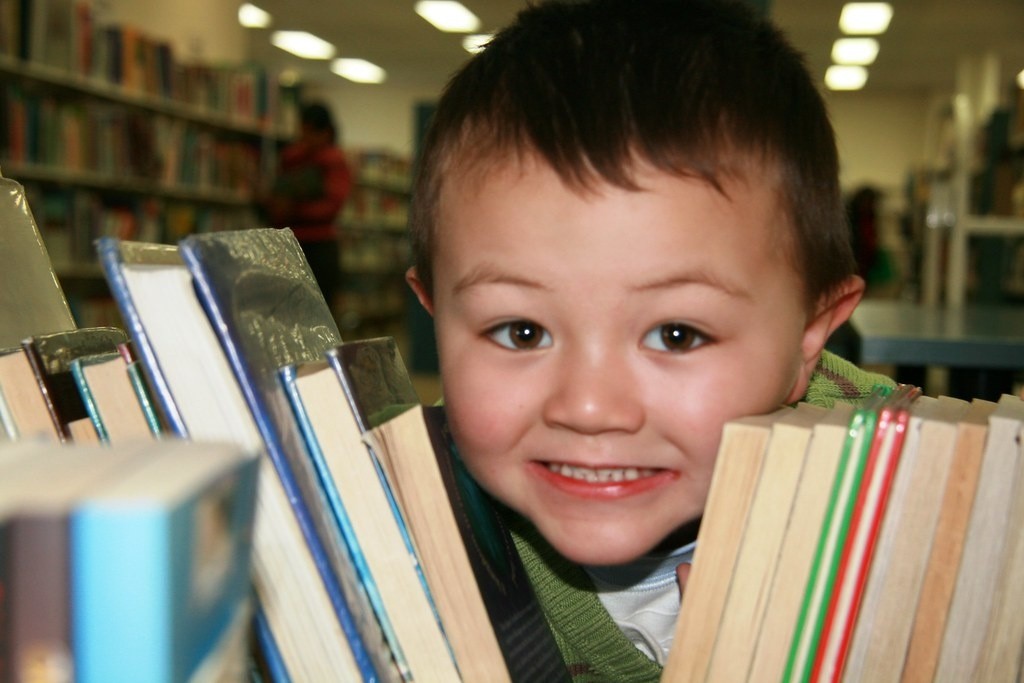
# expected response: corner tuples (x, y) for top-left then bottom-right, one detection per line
(848, 297), (1023, 397)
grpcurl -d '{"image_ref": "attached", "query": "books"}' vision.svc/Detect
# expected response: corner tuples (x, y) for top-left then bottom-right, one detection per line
(0, 1), (574, 683)
(661, 387), (1024, 683)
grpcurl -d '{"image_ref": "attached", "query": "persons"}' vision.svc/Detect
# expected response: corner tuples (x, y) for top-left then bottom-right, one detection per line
(266, 103), (354, 303)
(961, 123), (1013, 304)
(409, 0), (900, 683)
(846, 183), (899, 301)
